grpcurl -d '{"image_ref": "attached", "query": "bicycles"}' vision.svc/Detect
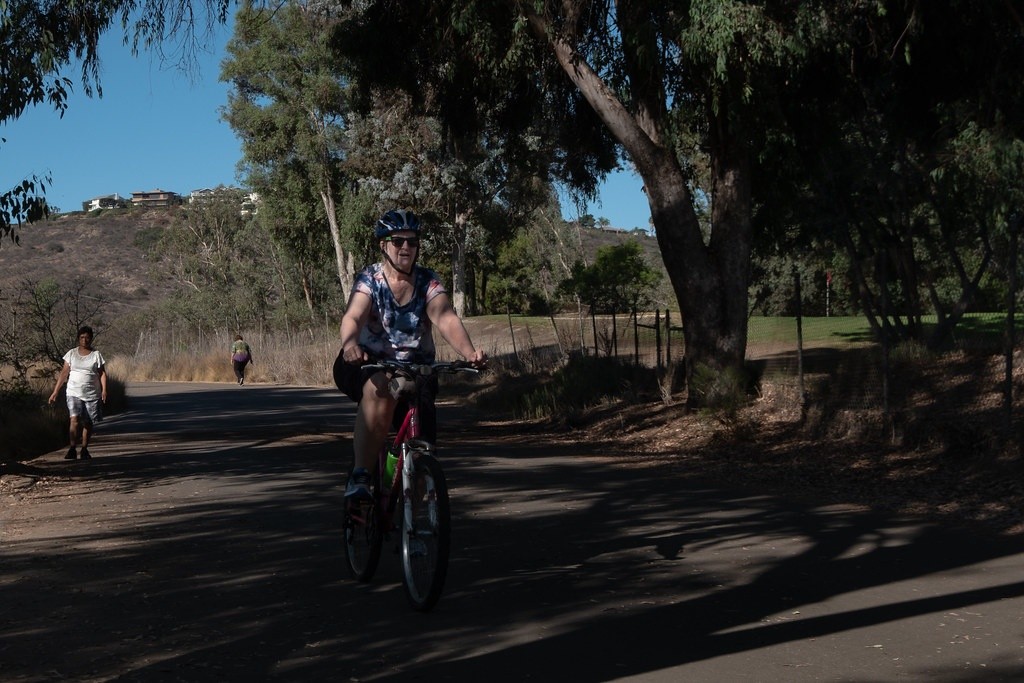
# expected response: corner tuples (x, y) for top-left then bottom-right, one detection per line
(339, 359), (490, 614)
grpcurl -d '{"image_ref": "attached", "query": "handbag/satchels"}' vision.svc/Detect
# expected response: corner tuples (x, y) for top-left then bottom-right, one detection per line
(332, 348), (363, 399)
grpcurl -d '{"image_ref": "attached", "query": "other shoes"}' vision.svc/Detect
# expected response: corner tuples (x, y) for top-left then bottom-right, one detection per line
(65, 448), (76, 460)
(80, 449), (92, 460)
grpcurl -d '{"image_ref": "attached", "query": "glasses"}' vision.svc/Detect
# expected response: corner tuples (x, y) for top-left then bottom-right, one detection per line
(384, 237), (421, 247)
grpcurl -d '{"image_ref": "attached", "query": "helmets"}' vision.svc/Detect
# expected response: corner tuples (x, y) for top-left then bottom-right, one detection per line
(375, 210), (422, 243)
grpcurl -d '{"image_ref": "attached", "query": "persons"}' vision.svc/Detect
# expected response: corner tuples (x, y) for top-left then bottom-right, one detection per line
(230, 334), (253, 386)
(48, 326), (107, 458)
(333, 208), (488, 561)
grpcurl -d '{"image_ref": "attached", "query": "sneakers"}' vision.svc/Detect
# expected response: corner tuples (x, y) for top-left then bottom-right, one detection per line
(407, 539), (427, 558)
(344, 466), (373, 502)
